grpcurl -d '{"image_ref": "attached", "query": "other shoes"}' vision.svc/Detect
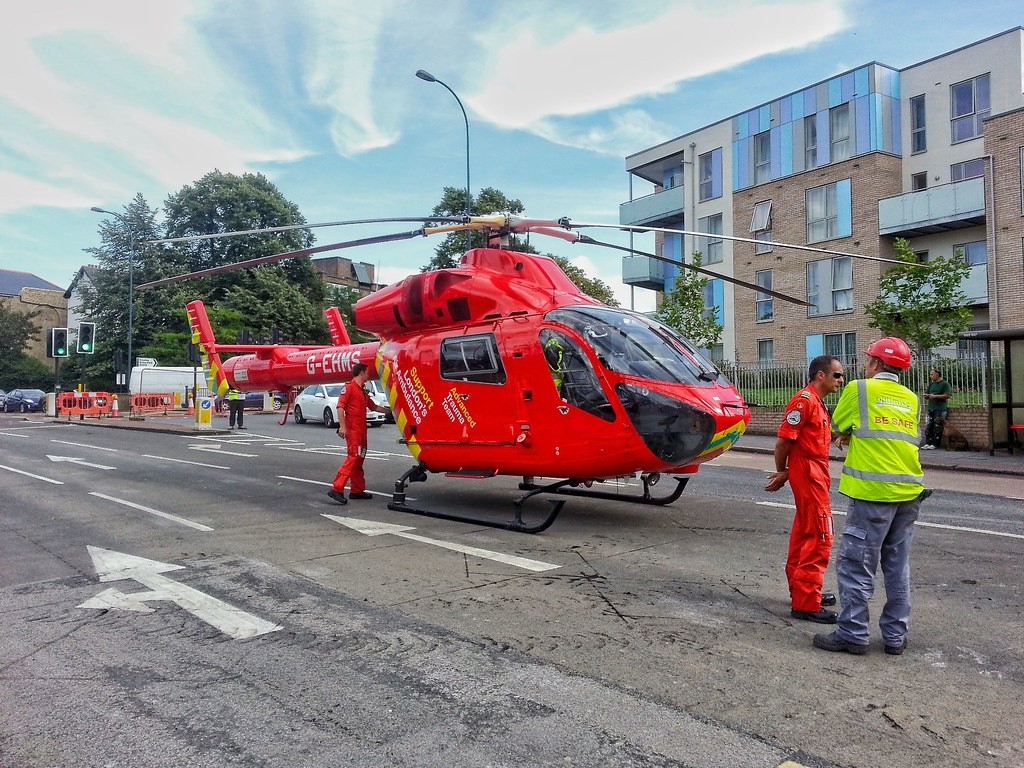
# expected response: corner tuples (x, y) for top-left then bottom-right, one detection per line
(228, 426), (234, 429)
(238, 425), (247, 429)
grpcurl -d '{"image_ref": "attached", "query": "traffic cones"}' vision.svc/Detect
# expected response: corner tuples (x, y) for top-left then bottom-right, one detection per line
(210, 392), (217, 415)
(108, 398), (123, 418)
(186, 397), (196, 415)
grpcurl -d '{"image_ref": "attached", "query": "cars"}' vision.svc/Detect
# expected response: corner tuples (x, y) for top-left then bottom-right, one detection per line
(293, 378), (394, 427)
(215, 391), (286, 411)
(0, 388), (107, 413)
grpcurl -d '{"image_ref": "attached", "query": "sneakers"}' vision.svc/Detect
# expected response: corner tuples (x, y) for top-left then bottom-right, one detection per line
(883, 634), (907, 656)
(921, 444), (929, 450)
(927, 445), (936, 450)
(811, 631), (870, 654)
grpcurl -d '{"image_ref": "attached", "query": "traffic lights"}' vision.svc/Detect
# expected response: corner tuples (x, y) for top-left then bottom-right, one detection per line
(273, 328), (283, 345)
(52, 327), (68, 357)
(243, 329), (253, 344)
(77, 323), (95, 353)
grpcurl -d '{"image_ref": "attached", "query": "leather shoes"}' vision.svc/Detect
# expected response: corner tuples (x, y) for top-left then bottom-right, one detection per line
(821, 592), (836, 607)
(327, 490), (347, 504)
(791, 604), (838, 625)
(349, 492), (372, 500)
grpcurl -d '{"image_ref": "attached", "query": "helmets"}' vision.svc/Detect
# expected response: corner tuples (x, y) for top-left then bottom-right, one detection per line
(862, 337), (911, 370)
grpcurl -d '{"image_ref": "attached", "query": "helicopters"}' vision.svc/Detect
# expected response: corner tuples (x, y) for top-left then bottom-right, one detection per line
(134, 210), (934, 534)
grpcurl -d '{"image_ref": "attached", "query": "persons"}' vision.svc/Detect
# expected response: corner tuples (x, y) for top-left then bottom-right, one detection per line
(764, 354), (844, 625)
(228, 389), (247, 430)
(814, 336), (924, 654)
(921, 369), (951, 450)
(327, 364), (391, 505)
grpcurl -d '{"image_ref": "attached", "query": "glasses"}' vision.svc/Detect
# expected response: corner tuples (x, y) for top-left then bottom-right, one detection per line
(823, 371), (843, 379)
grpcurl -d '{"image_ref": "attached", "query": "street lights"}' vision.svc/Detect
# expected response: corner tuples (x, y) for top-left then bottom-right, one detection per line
(418, 68), (470, 249)
(90, 207), (133, 393)
